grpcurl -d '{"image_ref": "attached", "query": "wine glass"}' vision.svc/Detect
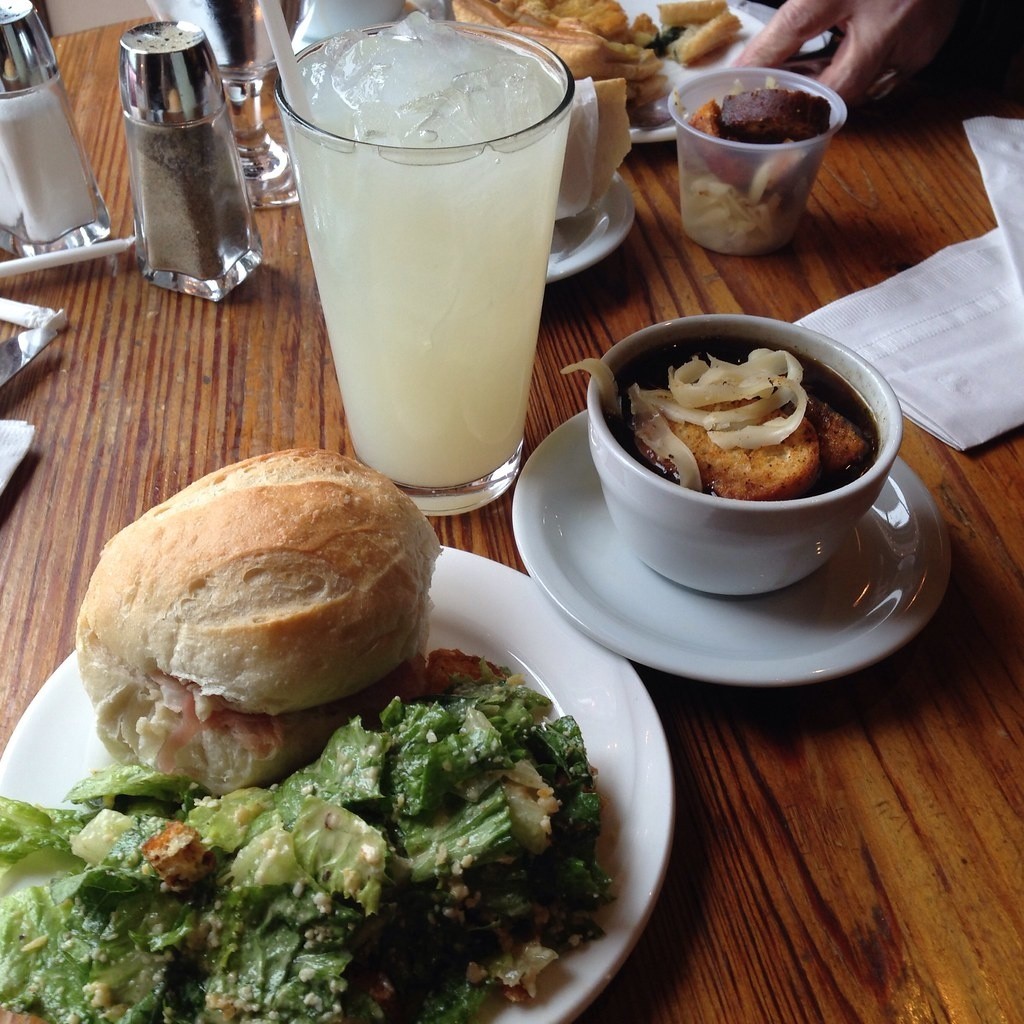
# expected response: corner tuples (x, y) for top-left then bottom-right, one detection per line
(146, 0), (305, 207)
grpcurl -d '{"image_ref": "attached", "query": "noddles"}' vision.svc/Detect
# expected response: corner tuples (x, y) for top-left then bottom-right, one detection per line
(560, 349), (808, 491)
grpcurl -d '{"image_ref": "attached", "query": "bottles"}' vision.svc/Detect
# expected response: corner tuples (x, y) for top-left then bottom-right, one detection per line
(120, 21), (262, 301)
(0, 0), (112, 258)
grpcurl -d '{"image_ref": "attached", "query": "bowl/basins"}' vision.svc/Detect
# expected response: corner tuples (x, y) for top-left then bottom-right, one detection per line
(587, 314), (901, 595)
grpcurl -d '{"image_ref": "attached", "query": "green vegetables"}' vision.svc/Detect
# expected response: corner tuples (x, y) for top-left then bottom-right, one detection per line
(0, 666), (619, 1024)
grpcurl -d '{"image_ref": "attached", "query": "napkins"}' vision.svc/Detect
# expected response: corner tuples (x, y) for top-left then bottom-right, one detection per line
(0, 419), (35, 499)
(787, 117), (1024, 453)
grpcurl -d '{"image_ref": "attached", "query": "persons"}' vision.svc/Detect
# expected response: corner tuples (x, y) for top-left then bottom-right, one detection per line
(738, 0), (976, 105)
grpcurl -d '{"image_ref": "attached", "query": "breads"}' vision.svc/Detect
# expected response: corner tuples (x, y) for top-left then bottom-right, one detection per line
(79, 446), (442, 795)
(451, 1), (832, 222)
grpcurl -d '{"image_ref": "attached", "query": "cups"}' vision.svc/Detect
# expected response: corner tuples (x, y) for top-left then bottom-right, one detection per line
(274, 23), (575, 517)
(666, 67), (848, 257)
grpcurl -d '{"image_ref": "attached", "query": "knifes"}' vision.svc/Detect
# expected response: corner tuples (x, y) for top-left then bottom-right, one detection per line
(629, 67), (901, 128)
(0, 328), (57, 387)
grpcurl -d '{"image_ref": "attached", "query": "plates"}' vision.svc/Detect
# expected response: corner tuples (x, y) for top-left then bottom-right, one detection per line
(618, 0), (832, 143)
(546, 169), (632, 281)
(1, 544), (674, 1024)
(513, 408), (950, 685)
